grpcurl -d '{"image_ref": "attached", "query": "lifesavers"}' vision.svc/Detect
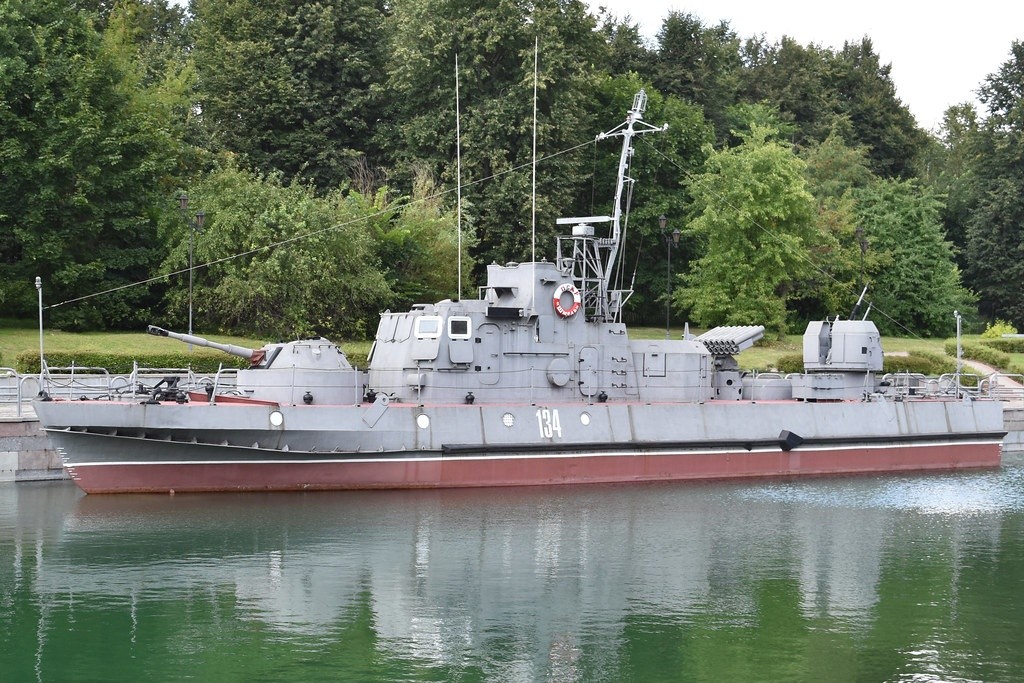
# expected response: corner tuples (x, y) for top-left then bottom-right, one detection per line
(552, 282), (581, 318)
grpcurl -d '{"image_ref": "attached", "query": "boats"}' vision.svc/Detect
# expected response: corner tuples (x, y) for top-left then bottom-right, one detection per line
(16, 35), (1010, 496)
